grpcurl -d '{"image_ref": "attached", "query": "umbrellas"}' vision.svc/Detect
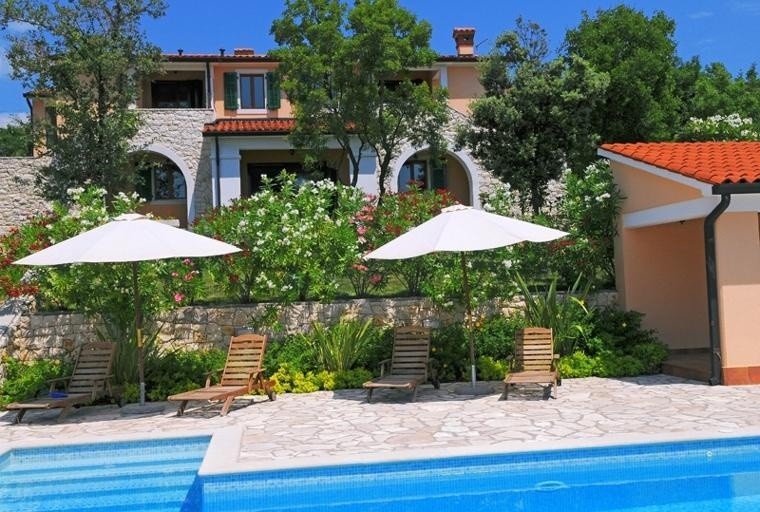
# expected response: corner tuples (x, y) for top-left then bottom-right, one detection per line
(361, 198), (572, 389)
(8, 206), (245, 404)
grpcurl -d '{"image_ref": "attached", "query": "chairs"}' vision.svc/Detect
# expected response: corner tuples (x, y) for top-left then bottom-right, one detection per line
(499, 327), (562, 400)
(6, 341), (123, 424)
(362, 326), (441, 401)
(168, 334), (276, 419)
